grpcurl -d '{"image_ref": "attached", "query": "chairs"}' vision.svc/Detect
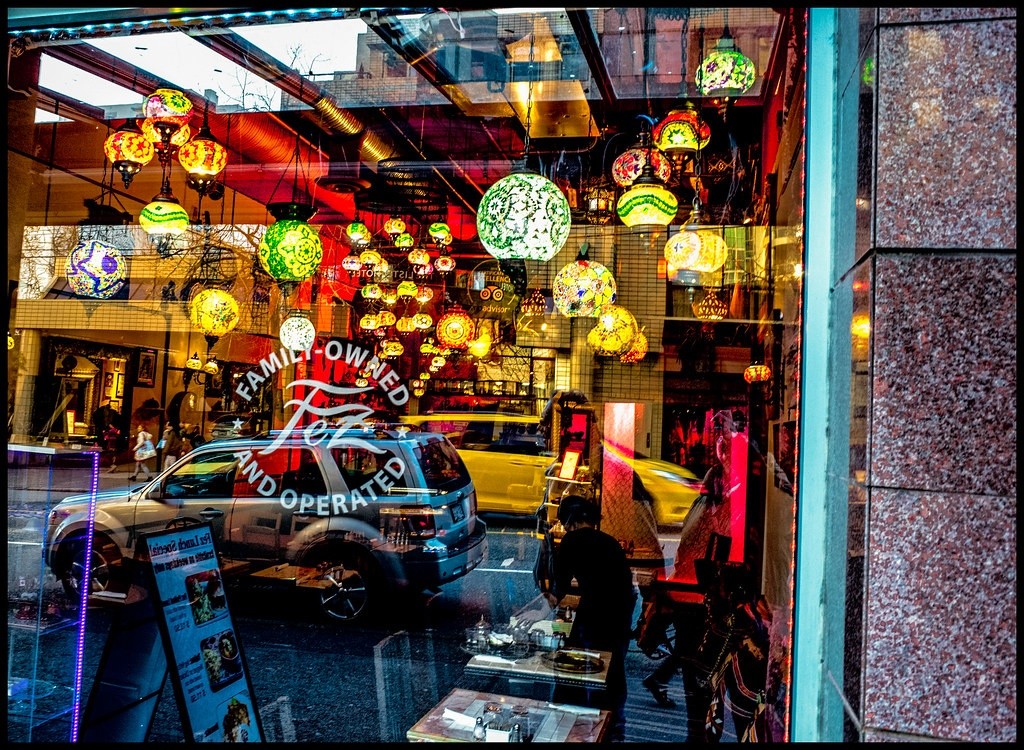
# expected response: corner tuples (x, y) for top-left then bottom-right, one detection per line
(374, 631), (416, 743)
(258, 694), (297, 742)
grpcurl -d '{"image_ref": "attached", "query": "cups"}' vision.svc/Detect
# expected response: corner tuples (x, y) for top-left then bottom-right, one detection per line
(483, 701), (502, 730)
(465, 627), (490, 654)
(510, 704), (530, 739)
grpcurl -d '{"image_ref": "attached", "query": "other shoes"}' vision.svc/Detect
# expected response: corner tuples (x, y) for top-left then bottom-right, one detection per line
(128, 476), (136, 481)
(643, 673), (676, 707)
(144, 476), (153, 482)
(107, 466), (120, 473)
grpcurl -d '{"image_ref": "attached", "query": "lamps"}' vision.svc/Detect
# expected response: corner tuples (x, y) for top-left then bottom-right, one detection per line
(552, 88), (648, 365)
(189, 289), (239, 351)
(342, 162), (491, 397)
(102, 86), (227, 258)
(279, 309), (315, 359)
(64, 121), (127, 327)
(257, 76), (322, 298)
(612, 9), (756, 339)
(480, 16), (571, 299)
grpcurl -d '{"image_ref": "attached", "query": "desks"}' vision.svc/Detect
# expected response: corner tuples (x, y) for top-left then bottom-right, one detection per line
(465, 645), (613, 701)
(406, 688), (610, 743)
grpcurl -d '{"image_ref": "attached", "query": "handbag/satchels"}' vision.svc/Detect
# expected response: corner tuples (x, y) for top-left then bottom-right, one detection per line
(156, 439), (167, 449)
(135, 440), (157, 461)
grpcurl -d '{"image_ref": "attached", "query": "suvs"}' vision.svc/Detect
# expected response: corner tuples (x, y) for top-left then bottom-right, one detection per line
(39, 426), (488, 634)
(400, 412), (703, 532)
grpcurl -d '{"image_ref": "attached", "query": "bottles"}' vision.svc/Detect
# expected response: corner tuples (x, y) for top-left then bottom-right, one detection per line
(473, 717), (485, 741)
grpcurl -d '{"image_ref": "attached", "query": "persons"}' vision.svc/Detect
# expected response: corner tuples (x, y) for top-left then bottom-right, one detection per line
(629, 406), (771, 746)
(102, 420), (208, 482)
(514, 493), (633, 741)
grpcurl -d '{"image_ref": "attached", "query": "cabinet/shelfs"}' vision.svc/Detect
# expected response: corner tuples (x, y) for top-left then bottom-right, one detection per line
(8, 442), (103, 742)
